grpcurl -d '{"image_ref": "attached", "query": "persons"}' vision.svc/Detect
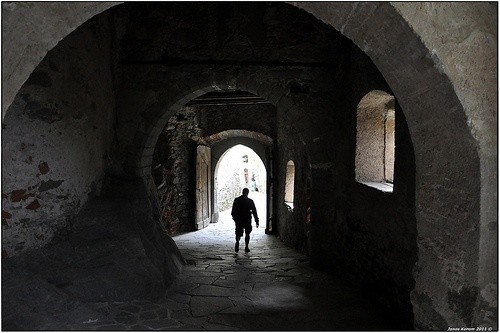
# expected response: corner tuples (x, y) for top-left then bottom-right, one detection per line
(231, 188), (259, 252)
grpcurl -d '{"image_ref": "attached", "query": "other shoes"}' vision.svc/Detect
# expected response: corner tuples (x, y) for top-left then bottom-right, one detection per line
(235, 242), (240, 252)
(245, 247), (250, 252)
